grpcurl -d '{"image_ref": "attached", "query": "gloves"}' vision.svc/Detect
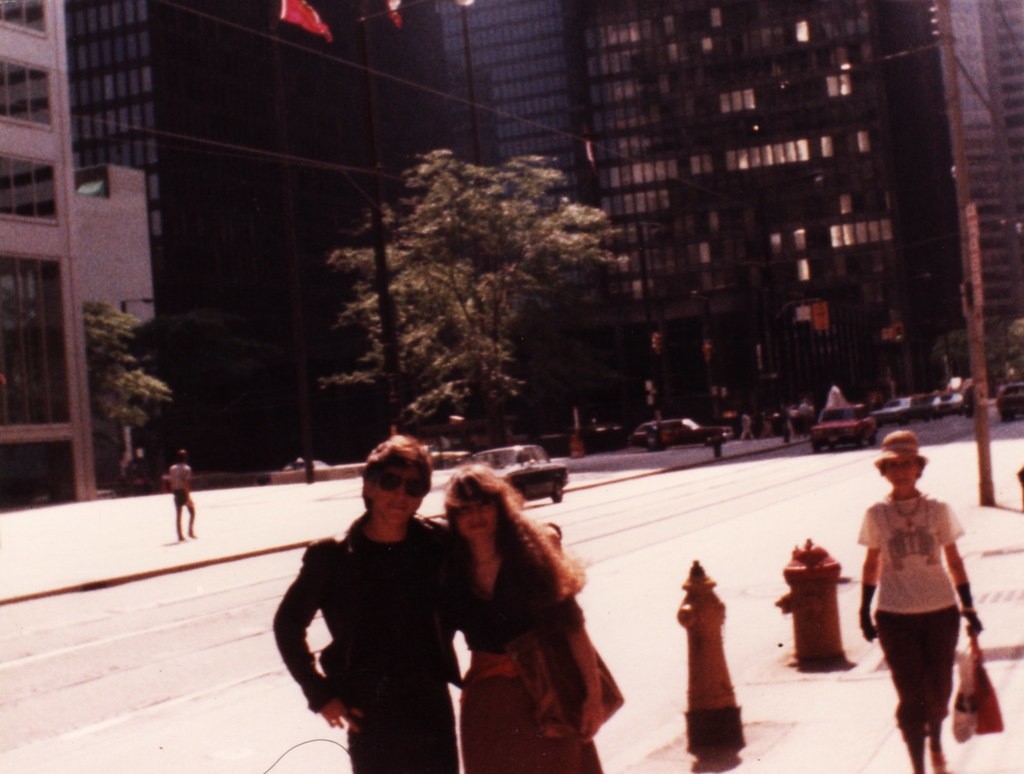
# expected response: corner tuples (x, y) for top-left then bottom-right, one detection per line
(955, 584), (983, 636)
(859, 583), (879, 642)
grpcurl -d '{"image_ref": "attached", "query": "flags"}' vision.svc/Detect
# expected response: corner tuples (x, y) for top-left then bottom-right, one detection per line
(277, 0), (410, 46)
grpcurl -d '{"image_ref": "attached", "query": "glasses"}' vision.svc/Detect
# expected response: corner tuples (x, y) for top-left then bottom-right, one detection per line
(378, 473), (428, 498)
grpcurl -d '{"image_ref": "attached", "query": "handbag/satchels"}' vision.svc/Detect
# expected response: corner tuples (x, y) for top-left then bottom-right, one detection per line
(953, 633), (1005, 743)
(506, 614), (624, 743)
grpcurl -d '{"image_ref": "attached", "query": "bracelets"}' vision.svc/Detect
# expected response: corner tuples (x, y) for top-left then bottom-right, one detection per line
(957, 608), (977, 616)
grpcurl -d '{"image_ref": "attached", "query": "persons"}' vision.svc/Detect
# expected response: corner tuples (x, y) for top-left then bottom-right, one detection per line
(737, 398), (825, 446)
(271, 438), (628, 774)
(858, 427), (987, 774)
(168, 448), (199, 543)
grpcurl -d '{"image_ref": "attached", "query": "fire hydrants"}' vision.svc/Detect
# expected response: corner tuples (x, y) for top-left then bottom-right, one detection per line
(676, 558), (746, 759)
(771, 536), (857, 662)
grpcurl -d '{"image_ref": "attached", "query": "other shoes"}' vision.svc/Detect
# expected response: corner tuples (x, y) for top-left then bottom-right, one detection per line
(930, 750), (946, 774)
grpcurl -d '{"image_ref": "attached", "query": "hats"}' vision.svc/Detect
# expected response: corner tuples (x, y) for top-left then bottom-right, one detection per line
(873, 430), (927, 470)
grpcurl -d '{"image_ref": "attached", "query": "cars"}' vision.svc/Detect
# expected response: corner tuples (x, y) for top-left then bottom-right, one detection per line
(466, 446), (569, 508)
(523, 380), (1024, 452)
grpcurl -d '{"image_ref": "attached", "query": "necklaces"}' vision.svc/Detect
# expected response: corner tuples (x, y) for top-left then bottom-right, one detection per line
(886, 491), (924, 528)
